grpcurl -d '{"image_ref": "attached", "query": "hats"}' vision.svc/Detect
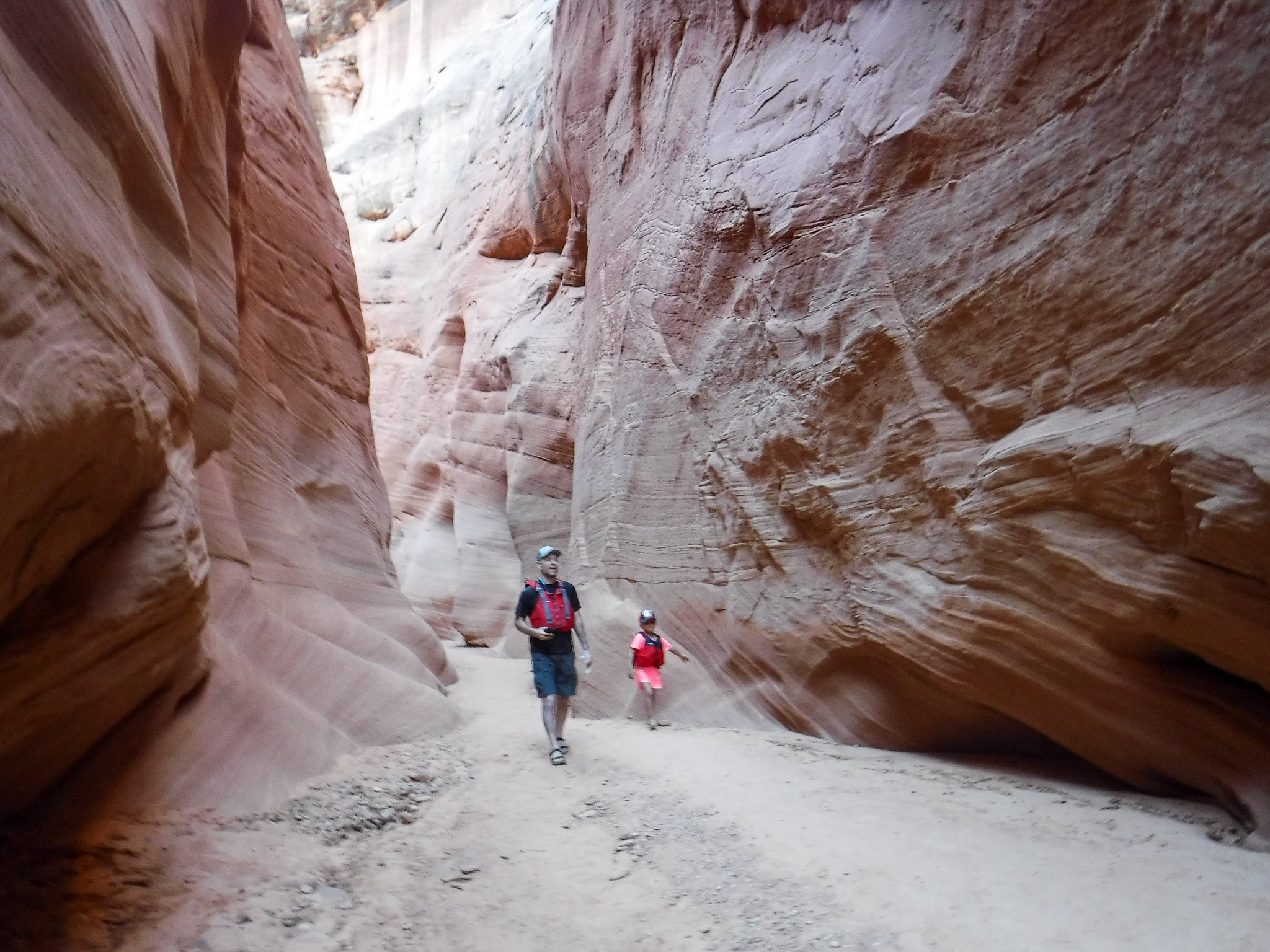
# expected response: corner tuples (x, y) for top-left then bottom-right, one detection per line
(639, 609), (656, 623)
(536, 545), (561, 561)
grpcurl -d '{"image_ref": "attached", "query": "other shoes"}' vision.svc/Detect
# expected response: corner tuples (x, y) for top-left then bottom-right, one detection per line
(650, 720), (657, 730)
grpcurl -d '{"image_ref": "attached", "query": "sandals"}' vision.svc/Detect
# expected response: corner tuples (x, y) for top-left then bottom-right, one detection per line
(556, 737), (568, 755)
(550, 748), (565, 765)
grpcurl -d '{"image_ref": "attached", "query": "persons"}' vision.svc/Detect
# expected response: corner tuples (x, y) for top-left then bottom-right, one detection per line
(514, 545), (594, 766)
(626, 609), (691, 730)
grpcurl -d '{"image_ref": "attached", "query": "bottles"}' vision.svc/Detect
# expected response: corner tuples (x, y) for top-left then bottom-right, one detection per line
(580, 653), (592, 673)
